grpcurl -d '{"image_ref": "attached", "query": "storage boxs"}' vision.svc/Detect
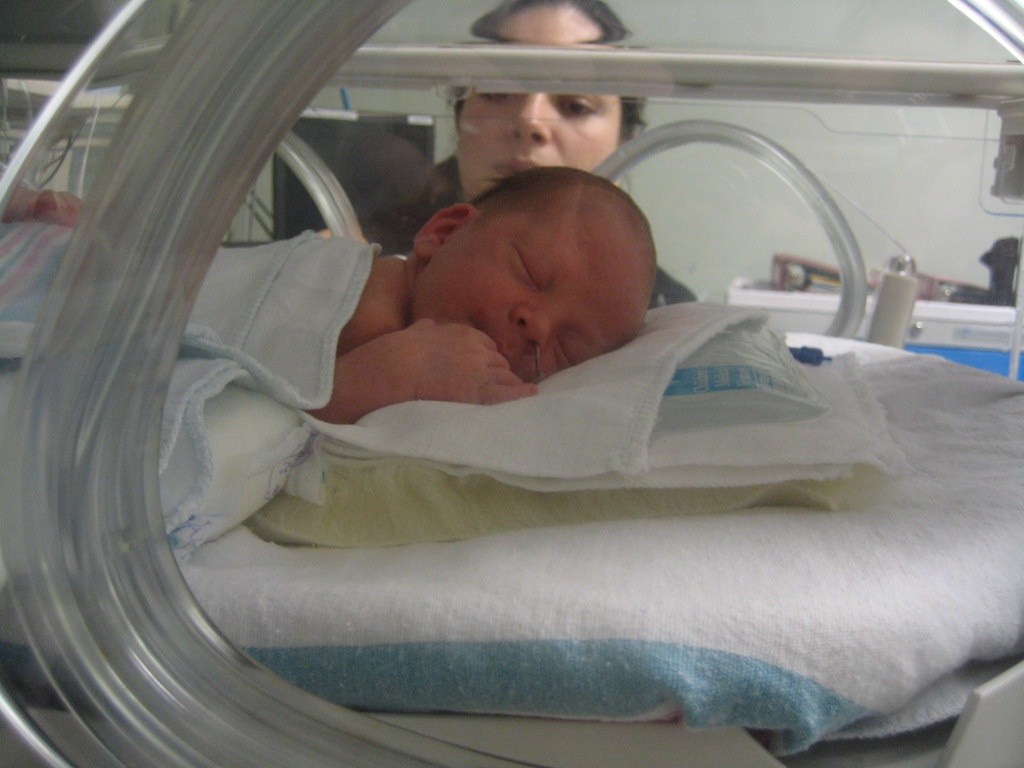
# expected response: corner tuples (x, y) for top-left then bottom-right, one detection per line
(728, 278), (1024, 382)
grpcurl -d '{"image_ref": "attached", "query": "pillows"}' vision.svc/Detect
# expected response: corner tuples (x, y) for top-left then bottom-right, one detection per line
(247, 318), (909, 542)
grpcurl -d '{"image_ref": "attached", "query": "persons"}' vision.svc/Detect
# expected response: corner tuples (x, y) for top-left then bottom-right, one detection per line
(0, 167), (656, 427)
(0, 0), (698, 304)
